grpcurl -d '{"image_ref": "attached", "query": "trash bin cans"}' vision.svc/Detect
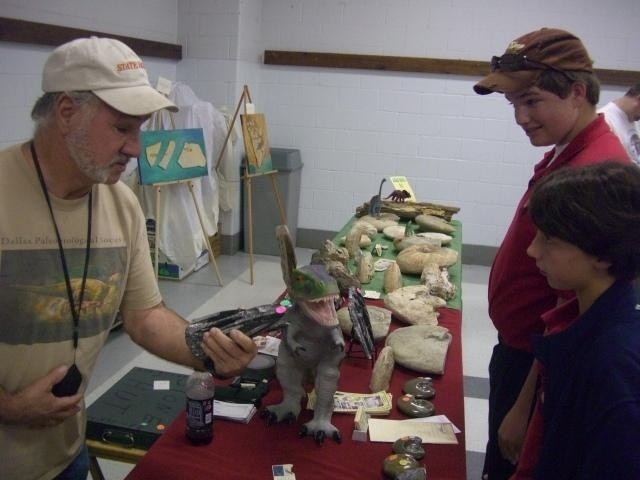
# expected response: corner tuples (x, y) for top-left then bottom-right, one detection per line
(240, 148), (304, 256)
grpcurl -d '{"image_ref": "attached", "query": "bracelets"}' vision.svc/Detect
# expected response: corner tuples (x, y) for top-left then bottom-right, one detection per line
(212, 369), (232, 380)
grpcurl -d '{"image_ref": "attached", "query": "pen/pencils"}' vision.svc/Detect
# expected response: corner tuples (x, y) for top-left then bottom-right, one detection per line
(229, 383), (254, 388)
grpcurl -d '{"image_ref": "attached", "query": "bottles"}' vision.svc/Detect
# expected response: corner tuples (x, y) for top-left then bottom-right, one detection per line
(186, 360), (214, 445)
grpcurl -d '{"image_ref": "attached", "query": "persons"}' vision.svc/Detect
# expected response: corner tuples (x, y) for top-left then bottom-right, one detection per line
(475, 27), (632, 480)
(1, 35), (258, 480)
(597, 83), (639, 166)
(509, 162), (640, 478)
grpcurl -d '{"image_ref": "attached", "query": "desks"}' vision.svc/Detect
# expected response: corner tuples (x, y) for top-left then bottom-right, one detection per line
(125, 209), (468, 480)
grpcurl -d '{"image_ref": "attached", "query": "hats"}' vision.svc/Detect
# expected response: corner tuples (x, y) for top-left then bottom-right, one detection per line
(41, 38), (178, 117)
(473, 28), (593, 94)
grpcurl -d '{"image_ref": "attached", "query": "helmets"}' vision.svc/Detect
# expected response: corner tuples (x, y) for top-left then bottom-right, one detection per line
(489, 56), (575, 82)
